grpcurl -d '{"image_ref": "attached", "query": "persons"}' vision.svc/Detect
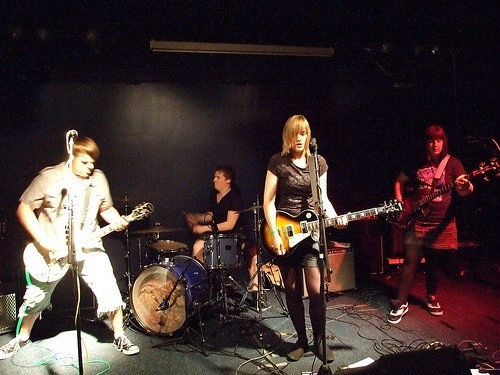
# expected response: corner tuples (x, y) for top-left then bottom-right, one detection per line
(0, 129), (140, 361)
(262, 115), (348, 363)
(182, 165), (259, 292)
(387, 123), (475, 325)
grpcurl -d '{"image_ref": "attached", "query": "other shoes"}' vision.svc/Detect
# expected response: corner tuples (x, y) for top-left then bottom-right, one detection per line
(287, 338), (308, 361)
(314, 341), (335, 362)
(253, 298), (272, 310)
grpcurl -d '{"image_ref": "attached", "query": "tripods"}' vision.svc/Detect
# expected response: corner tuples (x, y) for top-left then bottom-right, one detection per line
(119, 209), (289, 356)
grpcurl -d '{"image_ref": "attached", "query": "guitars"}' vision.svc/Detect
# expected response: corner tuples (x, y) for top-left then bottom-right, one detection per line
(22, 202), (154, 283)
(393, 157), (500, 234)
(260, 199), (406, 260)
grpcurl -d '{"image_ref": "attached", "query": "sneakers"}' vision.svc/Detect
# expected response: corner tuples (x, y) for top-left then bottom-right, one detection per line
(0, 337), (32, 359)
(425, 293), (443, 316)
(113, 336), (139, 356)
(388, 299), (409, 324)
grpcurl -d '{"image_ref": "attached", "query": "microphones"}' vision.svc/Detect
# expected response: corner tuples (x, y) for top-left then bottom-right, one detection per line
(310, 138), (316, 147)
(70, 130), (78, 137)
(212, 217), (219, 233)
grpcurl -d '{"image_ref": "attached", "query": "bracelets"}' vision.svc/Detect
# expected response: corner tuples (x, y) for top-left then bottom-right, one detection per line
(270, 230), (280, 236)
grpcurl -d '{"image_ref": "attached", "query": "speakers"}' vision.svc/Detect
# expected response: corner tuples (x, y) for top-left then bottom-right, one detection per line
(0, 281), (16, 335)
(299, 248), (356, 300)
(335, 346), (472, 375)
(346, 234), (384, 275)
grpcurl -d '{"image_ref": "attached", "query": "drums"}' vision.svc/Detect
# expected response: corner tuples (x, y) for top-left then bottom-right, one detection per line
(202, 234), (244, 270)
(129, 255), (213, 337)
(143, 240), (189, 269)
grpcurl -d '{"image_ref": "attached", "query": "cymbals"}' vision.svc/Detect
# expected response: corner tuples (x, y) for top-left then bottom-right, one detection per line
(232, 206), (262, 215)
(129, 226), (184, 234)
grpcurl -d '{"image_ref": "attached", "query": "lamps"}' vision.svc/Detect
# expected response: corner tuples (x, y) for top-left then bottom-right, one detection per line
(150, 38), (335, 57)
